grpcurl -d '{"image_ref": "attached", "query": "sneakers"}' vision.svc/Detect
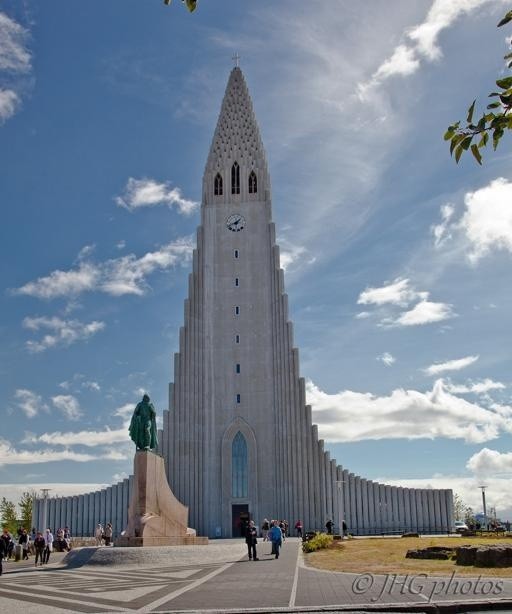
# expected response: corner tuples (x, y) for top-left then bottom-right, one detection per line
(249, 557), (259, 561)
(2, 555), (28, 561)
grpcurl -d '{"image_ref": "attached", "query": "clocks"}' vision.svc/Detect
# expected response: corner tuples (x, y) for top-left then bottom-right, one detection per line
(227, 214), (246, 232)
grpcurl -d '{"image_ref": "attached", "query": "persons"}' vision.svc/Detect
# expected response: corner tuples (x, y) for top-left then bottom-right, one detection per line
(103, 522), (113, 546)
(0, 523), (72, 576)
(342, 519), (349, 537)
(325, 519), (335, 533)
(244, 519), (260, 560)
(262, 516), (289, 542)
(128, 393), (159, 451)
(267, 519), (282, 559)
(294, 519), (303, 538)
(94, 523), (104, 546)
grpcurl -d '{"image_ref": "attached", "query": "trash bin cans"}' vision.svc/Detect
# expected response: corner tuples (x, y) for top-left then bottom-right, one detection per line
(15, 544), (23, 562)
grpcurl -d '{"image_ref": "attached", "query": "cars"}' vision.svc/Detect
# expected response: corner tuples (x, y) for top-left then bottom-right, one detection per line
(454, 520), (469, 534)
